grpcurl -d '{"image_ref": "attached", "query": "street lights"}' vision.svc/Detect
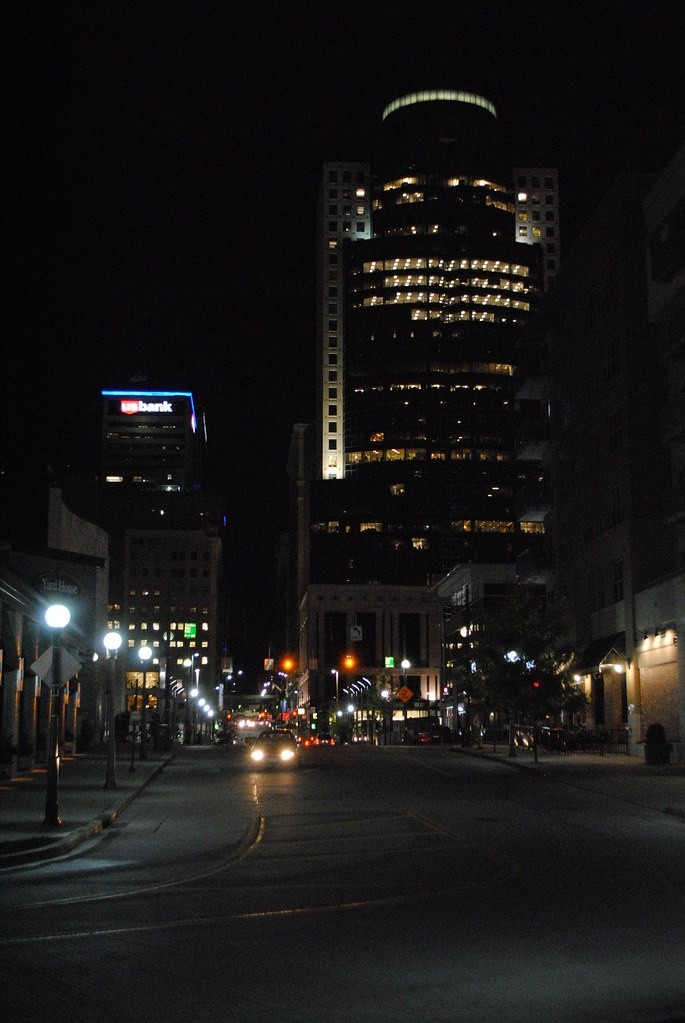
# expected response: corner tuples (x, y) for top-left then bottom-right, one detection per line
(131, 639), (159, 762)
(331, 669), (339, 740)
(35, 596), (74, 826)
(400, 658), (412, 744)
(95, 622), (130, 790)
(381, 689), (391, 745)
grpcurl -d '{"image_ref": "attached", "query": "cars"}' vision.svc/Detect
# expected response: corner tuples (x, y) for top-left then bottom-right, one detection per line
(211, 728), (336, 769)
(415, 725), (450, 744)
